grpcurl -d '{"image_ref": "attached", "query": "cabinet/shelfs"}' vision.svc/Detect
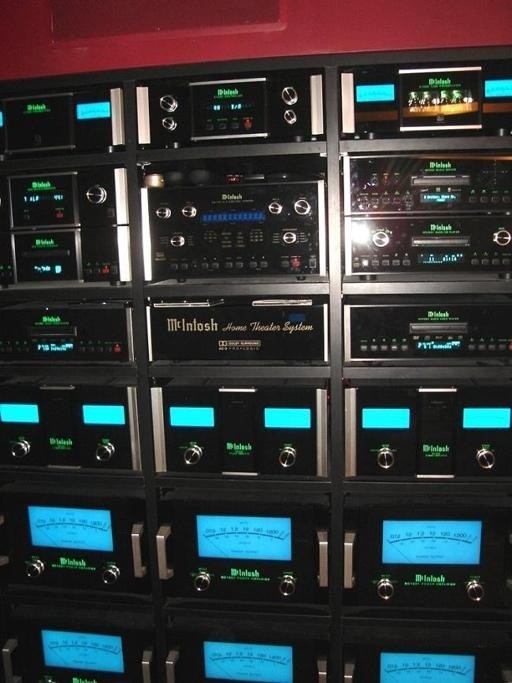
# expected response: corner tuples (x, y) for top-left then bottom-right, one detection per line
(0, 41), (510, 683)
